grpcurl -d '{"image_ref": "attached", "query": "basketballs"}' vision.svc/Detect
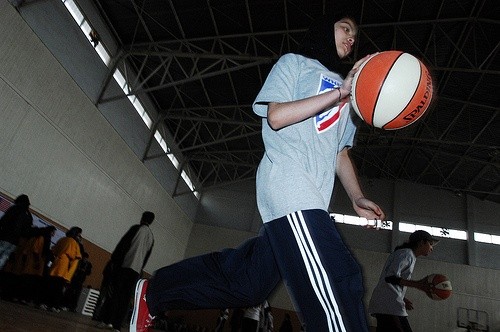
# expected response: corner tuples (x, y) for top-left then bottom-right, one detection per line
(424, 273), (453, 301)
(349, 50), (434, 131)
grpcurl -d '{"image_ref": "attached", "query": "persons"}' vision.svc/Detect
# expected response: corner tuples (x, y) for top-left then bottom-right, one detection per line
(368, 229), (439, 332)
(129, 10), (385, 332)
(0, 194), (154, 332)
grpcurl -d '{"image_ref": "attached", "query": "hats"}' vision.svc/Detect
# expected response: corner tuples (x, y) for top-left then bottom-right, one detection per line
(409, 230), (439, 242)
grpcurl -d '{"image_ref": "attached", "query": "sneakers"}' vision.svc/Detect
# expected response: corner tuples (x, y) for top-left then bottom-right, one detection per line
(129, 278), (157, 332)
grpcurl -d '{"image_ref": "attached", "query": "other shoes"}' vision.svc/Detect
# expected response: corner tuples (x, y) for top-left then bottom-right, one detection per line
(105, 323), (113, 328)
(94, 320), (105, 329)
(50, 306), (60, 312)
(38, 303), (47, 311)
